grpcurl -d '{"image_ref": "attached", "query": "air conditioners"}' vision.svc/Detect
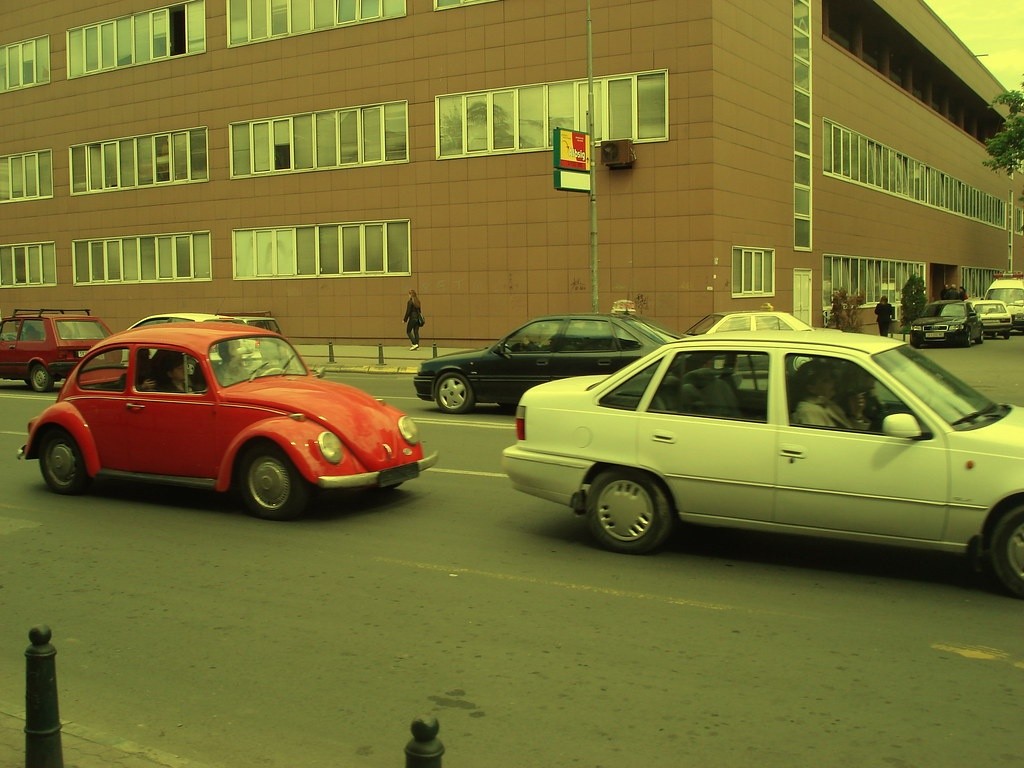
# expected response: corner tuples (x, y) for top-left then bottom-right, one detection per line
(602, 140), (633, 165)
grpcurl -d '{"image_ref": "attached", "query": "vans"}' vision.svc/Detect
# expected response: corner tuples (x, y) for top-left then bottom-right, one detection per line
(980, 272), (1024, 333)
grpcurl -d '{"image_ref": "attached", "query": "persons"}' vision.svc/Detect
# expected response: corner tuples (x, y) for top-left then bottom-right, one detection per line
(521, 322), (561, 350)
(401, 290), (421, 351)
(941, 284), (968, 301)
(114, 341), (250, 393)
(874, 295), (893, 337)
(25, 326), (41, 340)
(790, 359), (888, 433)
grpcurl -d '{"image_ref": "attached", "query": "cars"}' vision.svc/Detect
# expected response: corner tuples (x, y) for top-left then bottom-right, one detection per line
(908, 300), (984, 348)
(501, 330), (1024, 602)
(14, 320), (440, 521)
(120, 311), (285, 370)
(972, 300), (1013, 339)
(0, 306), (123, 394)
(680, 307), (844, 376)
(409, 310), (691, 415)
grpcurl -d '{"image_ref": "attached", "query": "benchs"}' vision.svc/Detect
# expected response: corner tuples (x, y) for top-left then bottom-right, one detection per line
(660, 372), (742, 416)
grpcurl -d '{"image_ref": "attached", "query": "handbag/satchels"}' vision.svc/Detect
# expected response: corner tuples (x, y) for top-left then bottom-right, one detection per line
(418, 313), (425, 327)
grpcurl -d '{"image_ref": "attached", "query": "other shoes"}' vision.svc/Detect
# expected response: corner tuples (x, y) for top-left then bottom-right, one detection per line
(409, 344), (418, 350)
(411, 345), (419, 350)
